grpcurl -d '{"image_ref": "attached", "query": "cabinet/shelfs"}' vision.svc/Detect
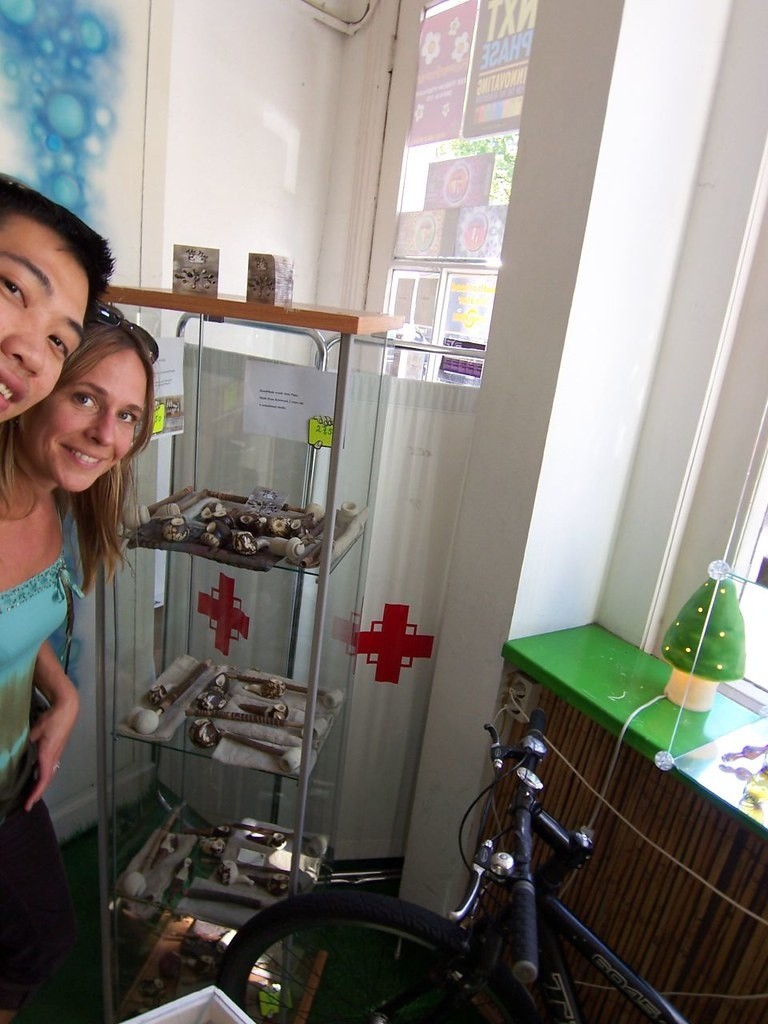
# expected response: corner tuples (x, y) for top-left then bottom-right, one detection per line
(92, 282), (387, 1024)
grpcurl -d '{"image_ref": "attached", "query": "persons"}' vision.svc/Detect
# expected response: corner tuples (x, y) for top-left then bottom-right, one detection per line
(2, 167), (114, 424)
(0, 302), (160, 1024)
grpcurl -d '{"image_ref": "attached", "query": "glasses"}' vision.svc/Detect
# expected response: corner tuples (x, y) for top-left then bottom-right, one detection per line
(94, 302), (160, 365)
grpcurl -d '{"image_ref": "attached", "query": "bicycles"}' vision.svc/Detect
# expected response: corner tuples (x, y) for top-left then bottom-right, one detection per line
(201, 714), (684, 1023)
